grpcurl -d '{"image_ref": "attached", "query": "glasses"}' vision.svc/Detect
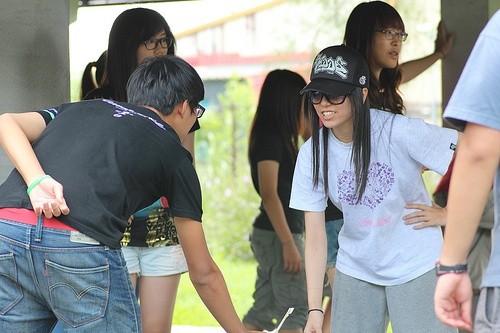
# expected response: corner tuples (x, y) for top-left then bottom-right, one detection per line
(138, 37), (173, 50)
(310, 91), (350, 105)
(374, 30), (408, 42)
(196, 103), (205, 118)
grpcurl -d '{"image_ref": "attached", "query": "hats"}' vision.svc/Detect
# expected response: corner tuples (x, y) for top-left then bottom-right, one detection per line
(299, 44), (369, 96)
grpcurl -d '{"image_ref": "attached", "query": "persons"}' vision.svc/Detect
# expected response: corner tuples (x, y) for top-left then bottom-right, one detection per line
(84, 8), (201, 333)
(80, 50), (107, 102)
(0, 55), (267, 333)
(243, 1), (500, 333)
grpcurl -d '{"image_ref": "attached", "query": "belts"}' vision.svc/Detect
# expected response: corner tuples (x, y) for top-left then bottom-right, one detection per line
(0, 207), (78, 231)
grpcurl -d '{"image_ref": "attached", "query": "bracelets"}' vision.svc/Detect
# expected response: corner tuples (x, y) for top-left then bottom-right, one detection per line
(26, 174), (51, 196)
(308, 309), (323, 314)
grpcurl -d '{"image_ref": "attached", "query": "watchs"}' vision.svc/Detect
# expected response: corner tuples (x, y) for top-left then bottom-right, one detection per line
(434, 260), (467, 277)
